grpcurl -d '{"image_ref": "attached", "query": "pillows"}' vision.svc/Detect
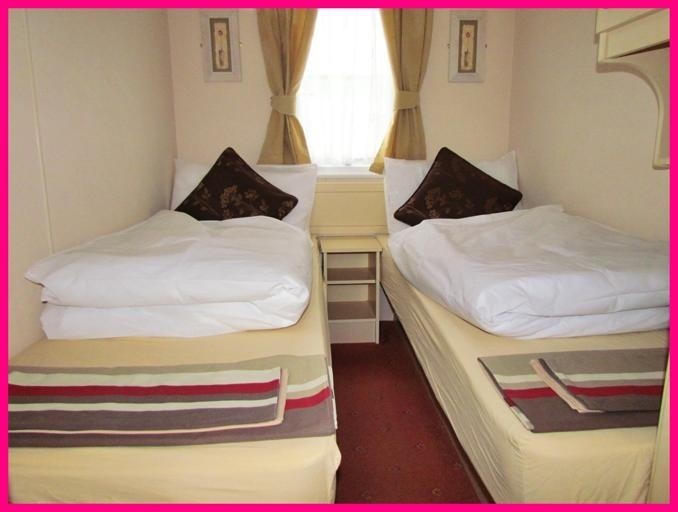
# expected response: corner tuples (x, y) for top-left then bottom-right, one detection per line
(383, 150), (519, 233)
(175, 149), (298, 220)
(394, 147), (522, 226)
(170, 157), (317, 246)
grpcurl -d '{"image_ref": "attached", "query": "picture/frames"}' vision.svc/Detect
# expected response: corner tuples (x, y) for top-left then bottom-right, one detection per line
(448, 11), (486, 83)
(200, 9), (241, 83)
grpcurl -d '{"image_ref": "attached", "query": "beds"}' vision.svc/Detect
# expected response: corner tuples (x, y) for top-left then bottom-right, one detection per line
(376, 232), (669, 504)
(8, 234), (342, 504)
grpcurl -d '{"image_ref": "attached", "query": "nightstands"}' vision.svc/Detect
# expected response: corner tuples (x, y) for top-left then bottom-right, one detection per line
(320, 236), (382, 345)
